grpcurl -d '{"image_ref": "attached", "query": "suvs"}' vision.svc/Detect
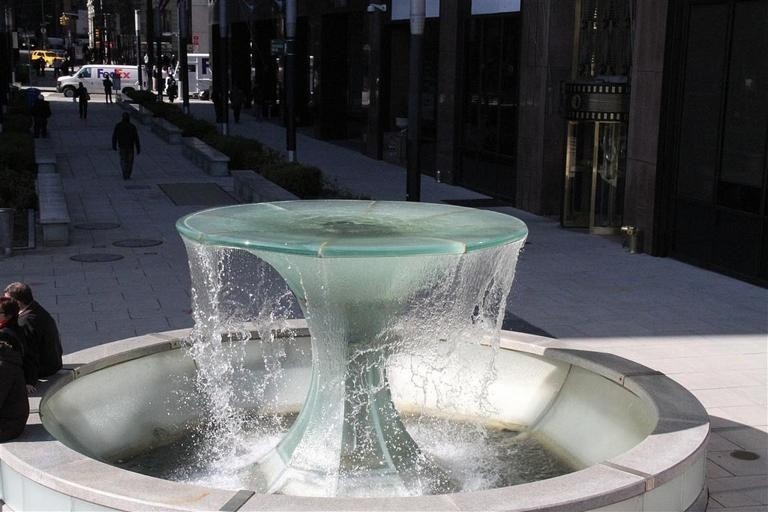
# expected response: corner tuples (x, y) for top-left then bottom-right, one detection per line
(31, 50), (65, 68)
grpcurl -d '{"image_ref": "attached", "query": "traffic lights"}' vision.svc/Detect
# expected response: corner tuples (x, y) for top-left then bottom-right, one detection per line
(59, 15), (64, 25)
(64, 16), (70, 25)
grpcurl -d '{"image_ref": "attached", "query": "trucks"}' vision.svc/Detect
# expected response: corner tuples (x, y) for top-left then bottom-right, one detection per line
(173, 53), (213, 100)
(55, 63), (148, 97)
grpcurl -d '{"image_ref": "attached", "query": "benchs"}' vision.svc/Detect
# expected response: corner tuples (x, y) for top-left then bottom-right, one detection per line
(116, 93), (134, 109)
(37, 173), (72, 247)
(34, 137), (57, 172)
(129, 103), (154, 125)
(151, 117), (183, 145)
(179, 137), (231, 176)
(230, 170), (301, 203)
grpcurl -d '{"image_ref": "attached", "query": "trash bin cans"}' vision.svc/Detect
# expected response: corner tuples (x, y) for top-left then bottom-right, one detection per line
(20, 63), (31, 86)
(0, 208), (16, 256)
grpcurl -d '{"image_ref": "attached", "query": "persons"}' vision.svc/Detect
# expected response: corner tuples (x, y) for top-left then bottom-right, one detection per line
(2, 281), (63, 377)
(38, 55), (178, 104)
(1, 297), (37, 395)
(250, 82), (267, 122)
(230, 86), (242, 123)
(110, 113), (141, 181)
(0, 342), (30, 443)
(75, 83), (90, 118)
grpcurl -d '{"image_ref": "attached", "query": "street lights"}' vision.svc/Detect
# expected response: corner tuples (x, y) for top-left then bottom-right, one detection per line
(143, 52), (153, 92)
(132, 8), (144, 91)
